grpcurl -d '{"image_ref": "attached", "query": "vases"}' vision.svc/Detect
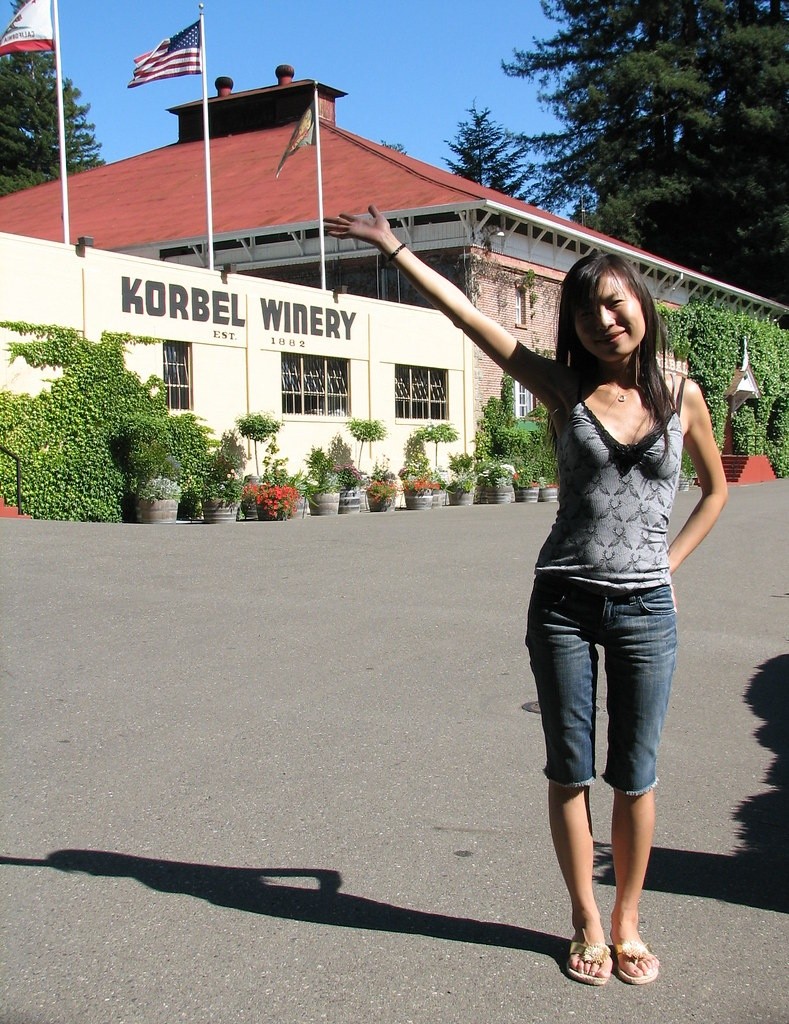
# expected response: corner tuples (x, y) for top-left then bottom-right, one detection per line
(366, 490), (386, 512)
(340, 489), (360, 513)
(404, 488), (433, 509)
(241, 499), (257, 519)
(255, 496), (287, 520)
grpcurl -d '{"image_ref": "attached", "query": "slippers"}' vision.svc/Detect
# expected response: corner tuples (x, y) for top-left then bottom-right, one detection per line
(616, 941), (660, 985)
(566, 940), (611, 985)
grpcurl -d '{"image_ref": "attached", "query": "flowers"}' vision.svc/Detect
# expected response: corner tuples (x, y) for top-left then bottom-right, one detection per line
(367, 480), (398, 502)
(404, 477), (439, 497)
(243, 482), (300, 516)
(333, 463), (363, 488)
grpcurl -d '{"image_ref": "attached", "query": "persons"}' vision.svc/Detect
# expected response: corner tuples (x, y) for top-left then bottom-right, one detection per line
(322, 205), (729, 988)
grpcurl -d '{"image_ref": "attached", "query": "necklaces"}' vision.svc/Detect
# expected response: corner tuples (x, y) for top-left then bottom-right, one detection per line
(592, 365), (634, 402)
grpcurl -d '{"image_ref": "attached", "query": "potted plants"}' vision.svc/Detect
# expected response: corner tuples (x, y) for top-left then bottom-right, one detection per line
(134, 476), (183, 523)
(433, 479), (446, 508)
(447, 479), (475, 505)
(198, 477), (243, 523)
(479, 465), (512, 504)
(512, 471), (540, 502)
(541, 475), (558, 502)
(303, 447), (339, 516)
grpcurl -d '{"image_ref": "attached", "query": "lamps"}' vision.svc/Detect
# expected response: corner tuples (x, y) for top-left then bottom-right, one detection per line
(490, 227), (505, 237)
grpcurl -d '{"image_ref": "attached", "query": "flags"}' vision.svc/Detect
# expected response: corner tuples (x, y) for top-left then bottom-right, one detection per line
(0, 0), (54, 55)
(127, 20), (203, 88)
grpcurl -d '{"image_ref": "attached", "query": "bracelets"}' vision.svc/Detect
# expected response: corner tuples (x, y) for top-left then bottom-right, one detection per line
(388, 244), (406, 261)
